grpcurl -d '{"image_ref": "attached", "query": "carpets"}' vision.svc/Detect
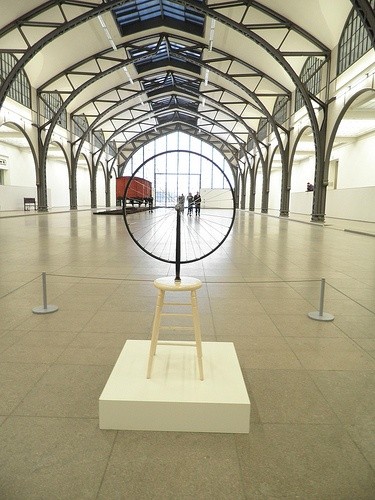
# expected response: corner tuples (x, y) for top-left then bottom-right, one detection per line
(93, 206), (157, 214)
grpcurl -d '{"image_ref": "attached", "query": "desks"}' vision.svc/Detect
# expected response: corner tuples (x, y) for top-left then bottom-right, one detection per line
(145, 277), (207, 380)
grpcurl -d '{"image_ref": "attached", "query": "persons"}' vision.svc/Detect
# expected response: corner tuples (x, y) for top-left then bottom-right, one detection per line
(187, 193), (194, 216)
(307, 183), (313, 191)
(148, 195), (153, 213)
(194, 191), (201, 216)
(180, 193), (185, 214)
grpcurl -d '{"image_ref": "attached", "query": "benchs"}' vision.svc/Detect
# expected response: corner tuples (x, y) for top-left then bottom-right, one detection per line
(24, 198), (36, 210)
